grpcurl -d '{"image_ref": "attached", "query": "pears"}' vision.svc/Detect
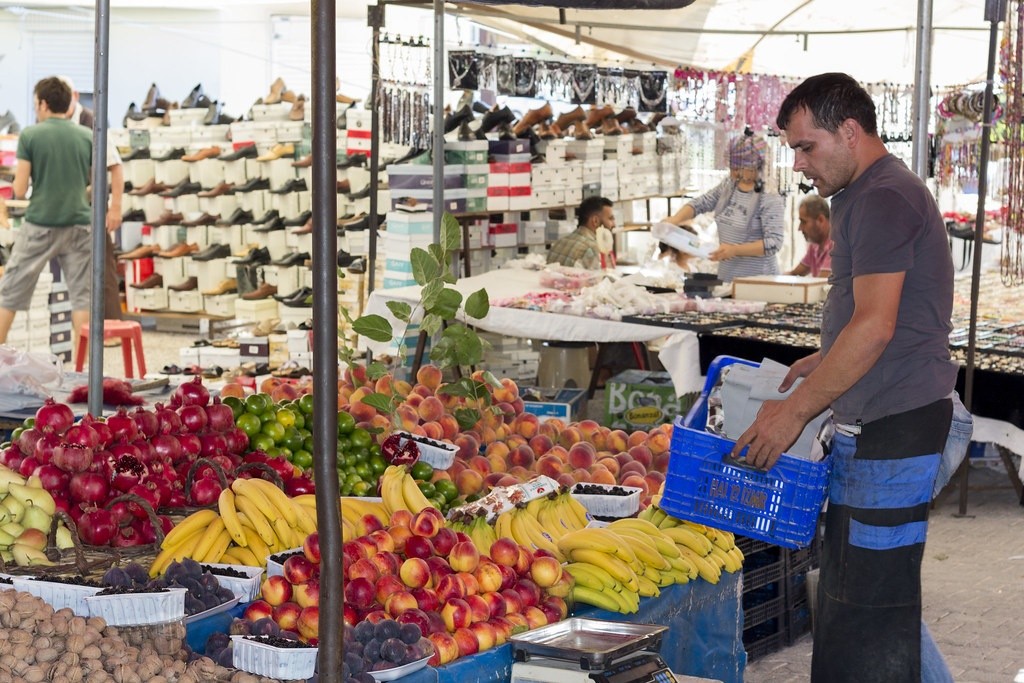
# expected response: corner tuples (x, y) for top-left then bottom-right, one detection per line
(0, 463), (71, 565)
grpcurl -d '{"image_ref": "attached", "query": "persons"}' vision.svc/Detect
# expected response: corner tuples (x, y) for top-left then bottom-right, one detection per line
(546, 196), (616, 270)
(789, 193), (833, 278)
(730, 72), (960, 683)
(658, 225), (699, 275)
(60, 75), (125, 348)
(660, 131), (785, 282)
(0, 77), (93, 362)
(921, 392), (974, 683)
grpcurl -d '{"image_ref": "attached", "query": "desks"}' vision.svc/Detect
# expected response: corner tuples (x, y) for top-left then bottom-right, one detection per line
(620, 279), (1024, 517)
(408, 259), (711, 400)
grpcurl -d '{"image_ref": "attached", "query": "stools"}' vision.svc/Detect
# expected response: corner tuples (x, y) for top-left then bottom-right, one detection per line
(74, 318), (143, 382)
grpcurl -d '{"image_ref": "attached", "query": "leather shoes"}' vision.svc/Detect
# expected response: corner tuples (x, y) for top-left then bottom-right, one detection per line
(0, 82), (391, 336)
(393, 102), (650, 210)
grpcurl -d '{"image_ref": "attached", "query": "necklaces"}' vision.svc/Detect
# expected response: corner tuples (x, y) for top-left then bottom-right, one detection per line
(379, 42), (983, 197)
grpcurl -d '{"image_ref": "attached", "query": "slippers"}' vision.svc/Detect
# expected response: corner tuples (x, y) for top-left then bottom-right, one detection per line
(160, 333), (313, 378)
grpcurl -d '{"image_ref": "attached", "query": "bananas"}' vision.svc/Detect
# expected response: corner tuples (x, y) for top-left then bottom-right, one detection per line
(148, 463), (434, 578)
(446, 485), (745, 614)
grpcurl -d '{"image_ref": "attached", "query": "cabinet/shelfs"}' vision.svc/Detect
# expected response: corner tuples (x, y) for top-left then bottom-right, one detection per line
(447, 195), (692, 255)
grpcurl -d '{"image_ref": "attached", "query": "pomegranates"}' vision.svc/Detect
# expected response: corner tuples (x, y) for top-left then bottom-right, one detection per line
(0, 375), (316, 549)
(379, 434), (422, 468)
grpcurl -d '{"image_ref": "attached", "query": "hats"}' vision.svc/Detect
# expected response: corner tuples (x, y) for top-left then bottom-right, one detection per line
(726, 131), (768, 171)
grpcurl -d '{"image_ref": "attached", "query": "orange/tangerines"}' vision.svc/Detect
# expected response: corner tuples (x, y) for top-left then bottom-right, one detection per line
(219, 392), (482, 518)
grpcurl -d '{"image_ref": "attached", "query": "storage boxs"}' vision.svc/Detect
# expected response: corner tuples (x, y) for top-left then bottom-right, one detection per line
(1, 104), (842, 682)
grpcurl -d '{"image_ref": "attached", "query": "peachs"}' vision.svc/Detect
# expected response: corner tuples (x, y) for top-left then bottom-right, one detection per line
(434, 410), (675, 513)
(212, 360), (525, 442)
(244, 504), (575, 665)
